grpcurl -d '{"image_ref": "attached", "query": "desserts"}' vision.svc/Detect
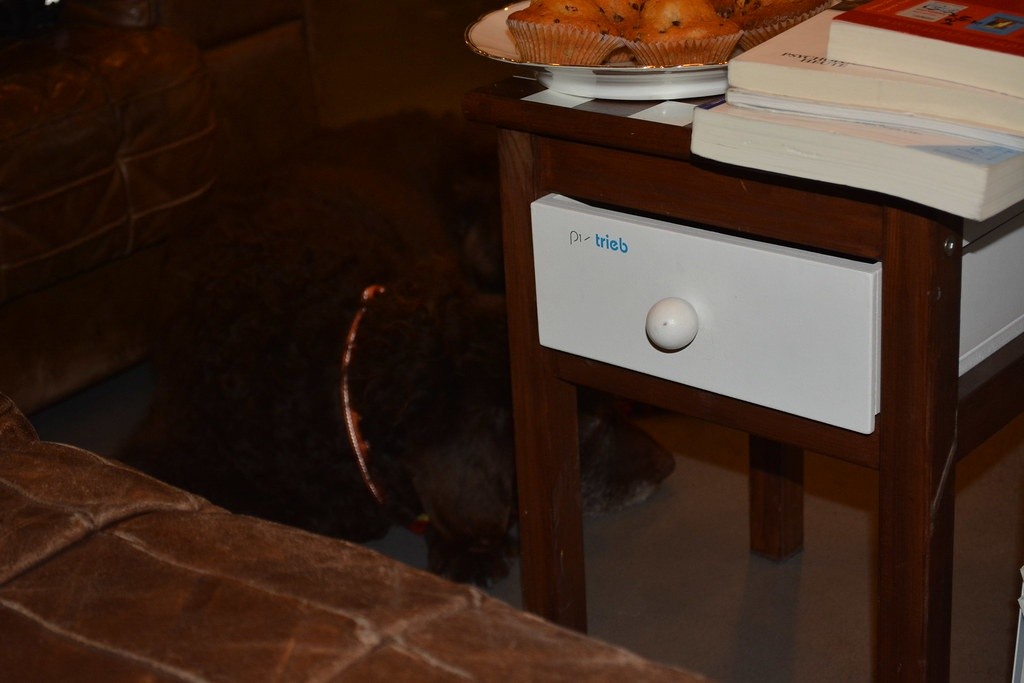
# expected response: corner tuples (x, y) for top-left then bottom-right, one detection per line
(506, 0), (834, 68)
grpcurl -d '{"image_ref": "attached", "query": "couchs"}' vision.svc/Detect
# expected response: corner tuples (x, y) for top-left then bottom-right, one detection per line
(0, 0), (654, 683)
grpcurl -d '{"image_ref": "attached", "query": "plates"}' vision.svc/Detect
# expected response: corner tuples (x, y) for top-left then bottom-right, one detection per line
(463, 0), (744, 100)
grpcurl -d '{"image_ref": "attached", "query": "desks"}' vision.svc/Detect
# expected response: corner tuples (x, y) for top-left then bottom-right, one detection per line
(459, 89), (956, 683)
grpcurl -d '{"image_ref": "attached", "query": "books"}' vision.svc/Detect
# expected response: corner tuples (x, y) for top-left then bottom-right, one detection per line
(689, 0), (1024, 223)
